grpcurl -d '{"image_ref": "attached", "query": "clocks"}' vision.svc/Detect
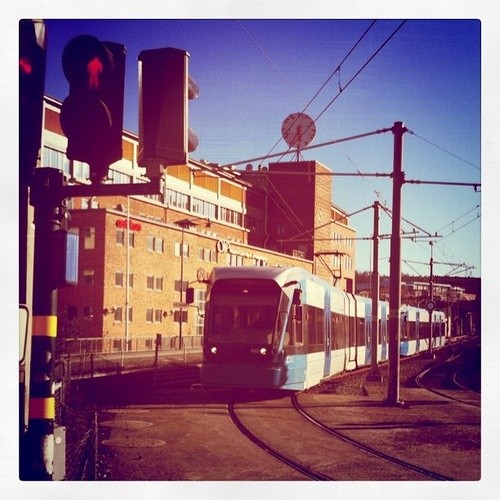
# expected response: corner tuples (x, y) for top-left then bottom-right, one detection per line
(282, 112), (316, 149)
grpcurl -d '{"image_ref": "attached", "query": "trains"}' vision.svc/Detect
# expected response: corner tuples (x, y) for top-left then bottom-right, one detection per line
(185, 266), (450, 393)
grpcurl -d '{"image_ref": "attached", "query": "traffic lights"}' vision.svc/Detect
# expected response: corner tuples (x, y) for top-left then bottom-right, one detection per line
(19, 19), (47, 174)
(137, 48), (202, 165)
(60, 32), (125, 165)
